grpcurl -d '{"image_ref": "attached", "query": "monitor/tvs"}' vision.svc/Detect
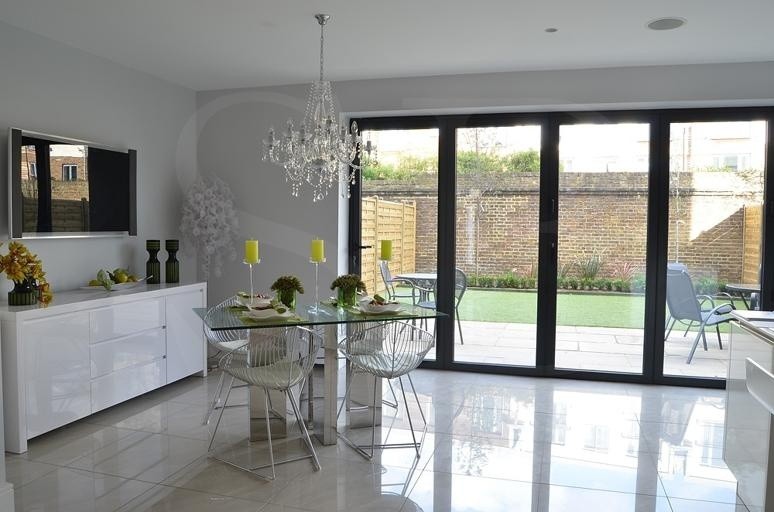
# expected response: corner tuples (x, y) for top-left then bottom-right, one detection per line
(8, 125), (138, 242)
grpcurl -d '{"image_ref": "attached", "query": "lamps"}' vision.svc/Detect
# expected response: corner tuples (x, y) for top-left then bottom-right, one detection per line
(259, 12), (380, 205)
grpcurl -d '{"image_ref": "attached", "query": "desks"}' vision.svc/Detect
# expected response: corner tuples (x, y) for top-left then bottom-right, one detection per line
(721, 309), (773, 512)
(1, 280), (209, 456)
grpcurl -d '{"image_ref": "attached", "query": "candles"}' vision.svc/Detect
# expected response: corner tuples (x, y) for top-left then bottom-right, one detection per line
(311, 236), (326, 262)
(381, 239), (393, 260)
(244, 237), (260, 264)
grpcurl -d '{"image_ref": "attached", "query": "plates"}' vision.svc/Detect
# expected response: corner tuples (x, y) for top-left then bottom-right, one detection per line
(348, 307), (407, 316)
(241, 311), (294, 322)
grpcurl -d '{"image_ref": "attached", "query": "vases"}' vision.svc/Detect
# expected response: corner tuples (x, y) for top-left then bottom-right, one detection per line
(8, 284), (38, 306)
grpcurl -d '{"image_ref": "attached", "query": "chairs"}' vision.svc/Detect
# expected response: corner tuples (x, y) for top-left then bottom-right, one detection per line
(664, 262), (763, 365)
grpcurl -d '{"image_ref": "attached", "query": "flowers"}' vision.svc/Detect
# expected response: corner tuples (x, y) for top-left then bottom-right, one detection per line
(1, 241), (54, 304)
(180, 173), (244, 281)
